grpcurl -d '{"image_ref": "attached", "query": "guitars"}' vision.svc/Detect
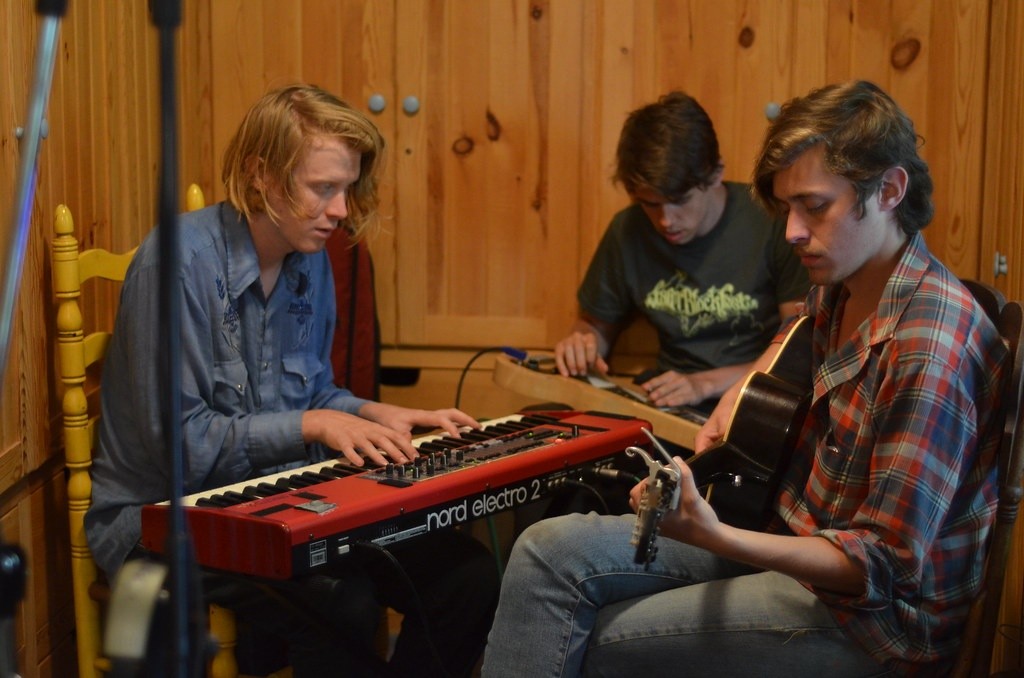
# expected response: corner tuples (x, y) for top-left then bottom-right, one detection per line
(625, 315), (822, 571)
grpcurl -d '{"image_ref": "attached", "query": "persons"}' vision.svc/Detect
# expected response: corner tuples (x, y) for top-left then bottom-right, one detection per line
(82, 82), (499, 678)
(479, 79), (1013, 677)
(553, 91), (813, 408)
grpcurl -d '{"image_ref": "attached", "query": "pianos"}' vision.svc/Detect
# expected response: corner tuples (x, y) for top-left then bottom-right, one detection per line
(140, 404), (656, 678)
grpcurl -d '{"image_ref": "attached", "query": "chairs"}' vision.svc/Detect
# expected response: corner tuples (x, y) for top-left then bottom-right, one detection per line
(53, 183), (240, 678)
(952, 276), (1023, 677)
